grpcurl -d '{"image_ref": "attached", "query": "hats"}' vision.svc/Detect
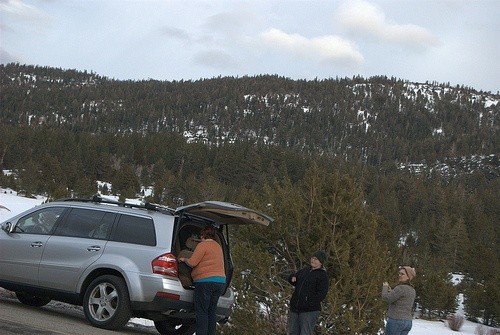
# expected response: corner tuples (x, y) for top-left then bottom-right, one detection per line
(313, 251), (327, 265)
(403, 266), (414, 281)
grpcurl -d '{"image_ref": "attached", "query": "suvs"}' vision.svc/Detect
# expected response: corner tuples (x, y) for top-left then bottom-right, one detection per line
(0, 193), (275, 330)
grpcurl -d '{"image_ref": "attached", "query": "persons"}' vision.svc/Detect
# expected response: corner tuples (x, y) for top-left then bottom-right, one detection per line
(382, 266), (417, 334)
(179, 227), (227, 335)
(286, 251), (329, 335)
